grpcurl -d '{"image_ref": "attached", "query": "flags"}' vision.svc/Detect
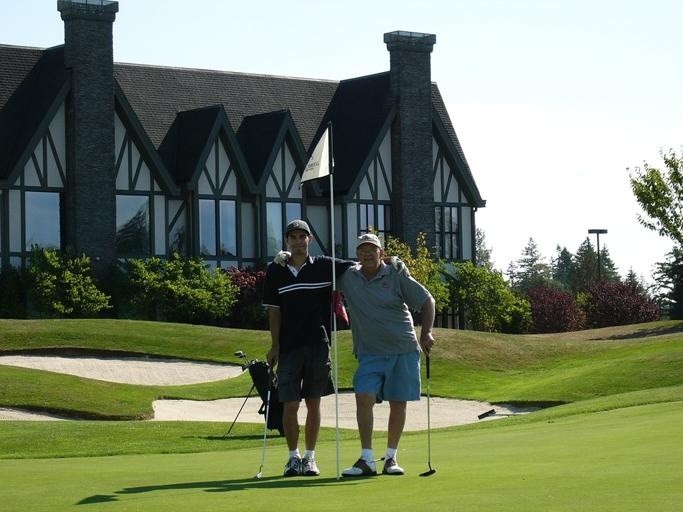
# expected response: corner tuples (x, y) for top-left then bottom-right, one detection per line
(299, 126), (329, 190)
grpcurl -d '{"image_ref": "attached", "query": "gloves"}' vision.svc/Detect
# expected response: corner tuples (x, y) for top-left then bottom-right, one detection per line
(390, 256), (410, 279)
(273, 249), (291, 268)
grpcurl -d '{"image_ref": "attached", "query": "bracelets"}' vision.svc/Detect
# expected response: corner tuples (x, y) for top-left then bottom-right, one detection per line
(421, 329), (431, 332)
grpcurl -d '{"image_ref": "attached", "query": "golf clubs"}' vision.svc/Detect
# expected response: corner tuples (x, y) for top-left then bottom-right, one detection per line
(255, 359), (272, 479)
(418, 355), (435, 476)
(223, 351), (286, 439)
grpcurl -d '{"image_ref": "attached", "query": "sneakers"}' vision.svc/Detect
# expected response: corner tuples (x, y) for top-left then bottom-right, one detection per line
(341, 457), (377, 475)
(283, 457), (301, 477)
(382, 458), (404, 475)
(302, 457), (320, 476)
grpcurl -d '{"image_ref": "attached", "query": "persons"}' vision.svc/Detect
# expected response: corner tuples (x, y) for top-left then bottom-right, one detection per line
(273, 234), (436, 478)
(262, 220), (411, 478)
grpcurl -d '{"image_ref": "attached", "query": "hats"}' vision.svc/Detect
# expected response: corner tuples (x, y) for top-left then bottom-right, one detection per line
(356, 234), (382, 251)
(285, 219), (311, 236)
